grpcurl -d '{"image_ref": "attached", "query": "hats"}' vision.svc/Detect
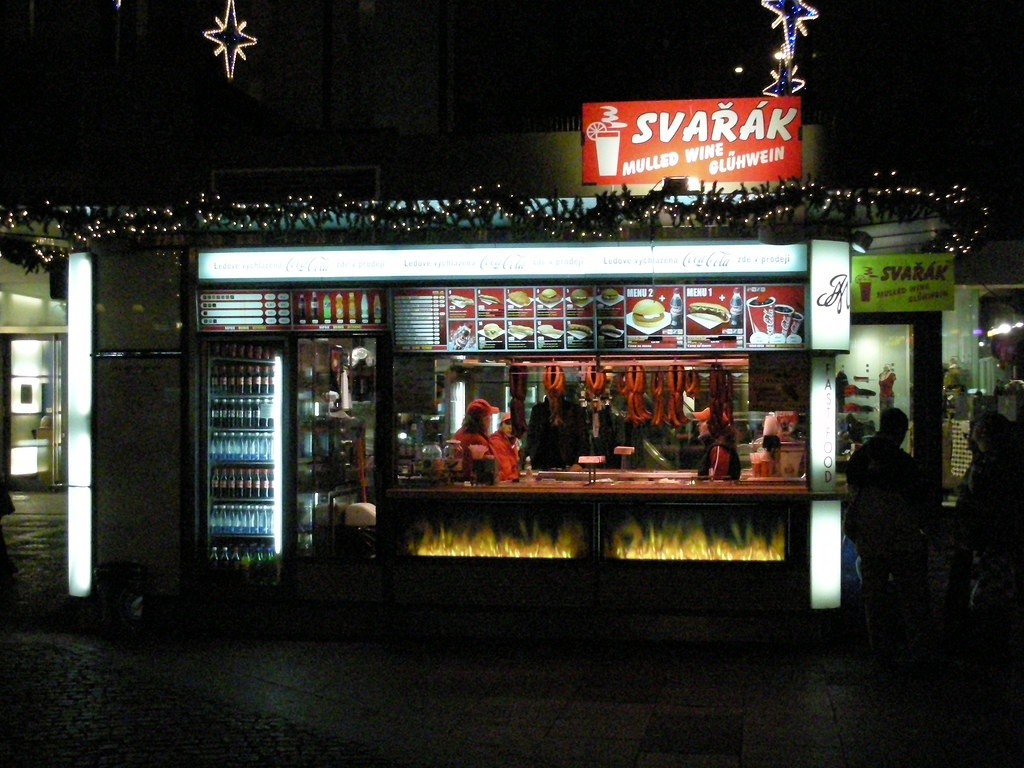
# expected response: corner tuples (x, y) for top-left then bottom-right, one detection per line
(692, 407), (729, 422)
(467, 399), (499, 415)
(502, 414), (511, 422)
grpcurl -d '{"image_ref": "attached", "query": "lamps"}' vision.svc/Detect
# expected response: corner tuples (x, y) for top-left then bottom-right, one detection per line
(662, 177), (690, 193)
(350, 337), (371, 363)
(849, 228), (874, 254)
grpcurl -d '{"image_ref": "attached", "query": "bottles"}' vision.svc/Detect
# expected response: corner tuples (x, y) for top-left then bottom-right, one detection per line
(323, 293), (331, 319)
(335, 291), (343, 318)
(360, 292), (369, 318)
(373, 294), (381, 318)
(298, 294), (306, 320)
(311, 291), (318, 319)
(731, 288), (743, 328)
(208, 342), (277, 566)
(670, 288), (683, 329)
(348, 292), (356, 318)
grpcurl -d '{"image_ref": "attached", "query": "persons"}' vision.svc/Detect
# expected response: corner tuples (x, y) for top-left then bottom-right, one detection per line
(844, 407), (1024, 692)
(450, 374), (742, 481)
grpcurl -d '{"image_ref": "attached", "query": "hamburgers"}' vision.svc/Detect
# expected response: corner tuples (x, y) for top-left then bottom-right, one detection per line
(483, 323), (500, 337)
(570, 289), (588, 304)
(569, 324), (592, 337)
(601, 288), (619, 303)
(633, 299), (665, 328)
(539, 288), (560, 303)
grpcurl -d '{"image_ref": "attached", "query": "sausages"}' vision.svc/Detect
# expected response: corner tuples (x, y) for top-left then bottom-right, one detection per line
(602, 328), (621, 335)
(509, 365), (734, 441)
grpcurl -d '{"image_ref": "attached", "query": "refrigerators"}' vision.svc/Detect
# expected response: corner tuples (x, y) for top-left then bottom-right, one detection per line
(197, 333), (392, 586)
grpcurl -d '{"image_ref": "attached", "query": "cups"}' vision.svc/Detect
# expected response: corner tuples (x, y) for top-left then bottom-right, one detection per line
(747, 296), (777, 334)
(860, 281), (872, 301)
(789, 312), (803, 335)
(774, 305), (794, 335)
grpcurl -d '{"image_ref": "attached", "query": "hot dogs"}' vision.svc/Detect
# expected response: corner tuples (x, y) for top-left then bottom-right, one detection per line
(689, 302), (730, 323)
(478, 295), (500, 305)
(449, 295), (474, 306)
